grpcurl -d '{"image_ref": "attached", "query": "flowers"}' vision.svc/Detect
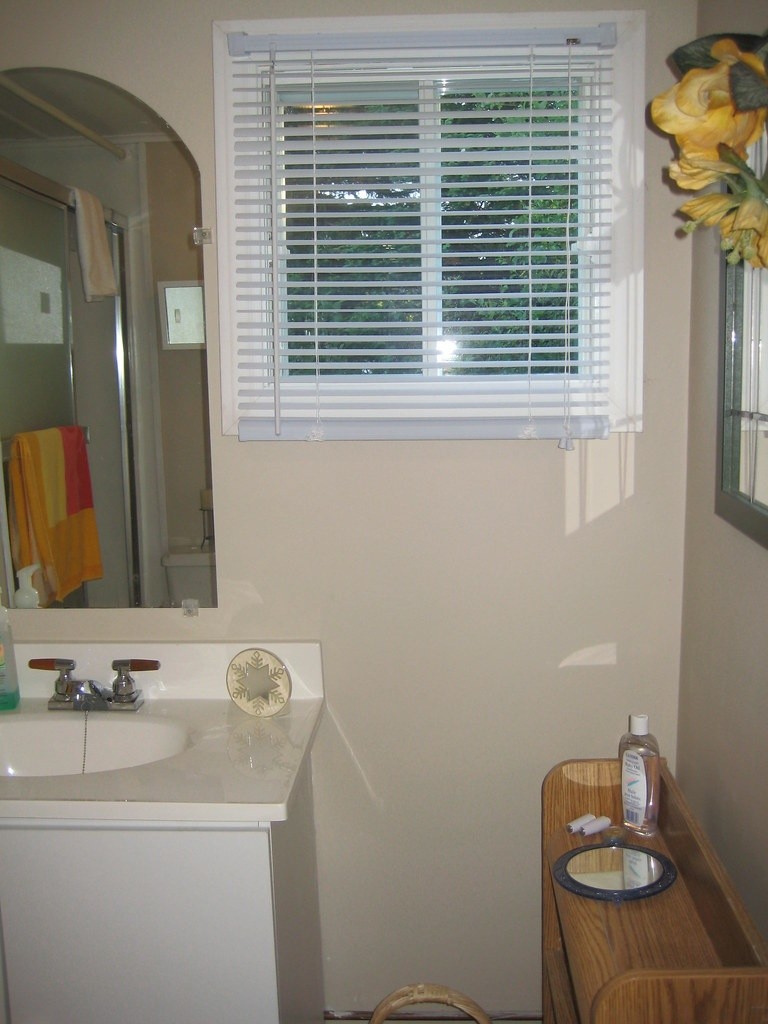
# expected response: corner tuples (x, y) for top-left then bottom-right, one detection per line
(648, 28), (768, 271)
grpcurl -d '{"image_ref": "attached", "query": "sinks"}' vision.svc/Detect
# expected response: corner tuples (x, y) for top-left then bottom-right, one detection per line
(0, 711), (205, 778)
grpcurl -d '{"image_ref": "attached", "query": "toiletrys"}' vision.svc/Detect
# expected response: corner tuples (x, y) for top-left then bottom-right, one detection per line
(618, 713), (660, 839)
(0, 585), (21, 711)
(11, 562), (45, 608)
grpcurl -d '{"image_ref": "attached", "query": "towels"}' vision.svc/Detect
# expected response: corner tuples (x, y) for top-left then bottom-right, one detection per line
(61, 184), (121, 303)
(7, 425), (104, 609)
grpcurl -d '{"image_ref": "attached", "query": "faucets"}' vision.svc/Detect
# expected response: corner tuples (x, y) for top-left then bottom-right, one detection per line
(72, 679), (110, 711)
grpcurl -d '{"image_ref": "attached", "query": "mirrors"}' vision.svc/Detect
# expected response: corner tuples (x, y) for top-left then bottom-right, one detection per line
(0, 64), (221, 612)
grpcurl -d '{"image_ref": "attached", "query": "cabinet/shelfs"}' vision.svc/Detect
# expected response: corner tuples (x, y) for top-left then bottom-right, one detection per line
(537, 752), (768, 1023)
